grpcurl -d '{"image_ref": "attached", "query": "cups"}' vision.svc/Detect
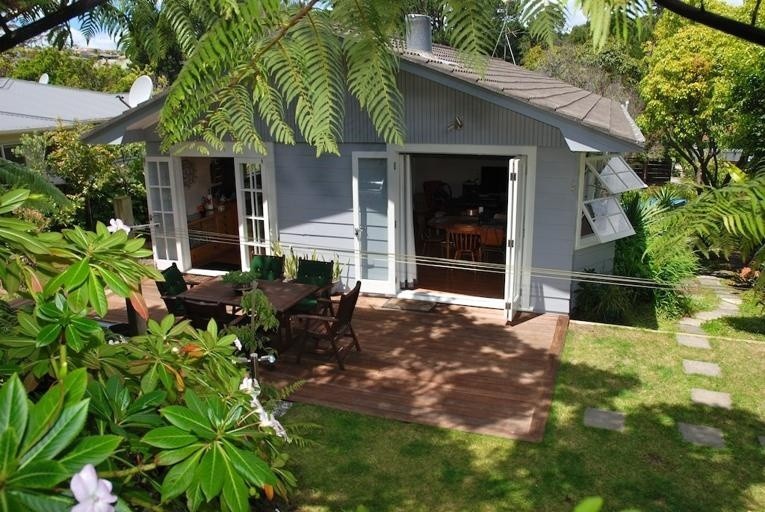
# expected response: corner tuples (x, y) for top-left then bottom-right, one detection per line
(479, 207), (483, 214)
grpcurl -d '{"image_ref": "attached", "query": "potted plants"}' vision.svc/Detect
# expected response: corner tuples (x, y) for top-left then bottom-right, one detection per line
(220, 271), (260, 294)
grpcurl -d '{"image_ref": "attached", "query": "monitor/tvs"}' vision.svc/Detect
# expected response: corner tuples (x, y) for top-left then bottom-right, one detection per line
(481, 166), (506, 193)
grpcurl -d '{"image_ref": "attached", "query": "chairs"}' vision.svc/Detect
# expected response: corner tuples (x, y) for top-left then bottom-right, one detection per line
(190, 298), (248, 340)
(412, 178), (506, 272)
(290, 279), (366, 371)
(248, 253), (286, 282)
(161, 293), (198, 327)
(156, 261), (199, 324)
(288, 257), (337, 324)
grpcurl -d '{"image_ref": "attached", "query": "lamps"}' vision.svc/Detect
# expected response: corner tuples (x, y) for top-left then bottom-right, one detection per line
(448, 111), (465, 134)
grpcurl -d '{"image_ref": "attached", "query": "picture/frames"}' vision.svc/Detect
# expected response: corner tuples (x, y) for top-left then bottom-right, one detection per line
(382, 296), (438, 312)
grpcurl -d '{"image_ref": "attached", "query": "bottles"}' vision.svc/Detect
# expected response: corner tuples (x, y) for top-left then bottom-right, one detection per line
(206, 188), (213, 210)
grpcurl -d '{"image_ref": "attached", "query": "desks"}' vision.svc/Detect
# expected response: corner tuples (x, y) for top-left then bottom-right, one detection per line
(176, 273), (319, 365)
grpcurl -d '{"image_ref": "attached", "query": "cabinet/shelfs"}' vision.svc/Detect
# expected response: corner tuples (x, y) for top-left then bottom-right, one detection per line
(210, 197), (238, 249)
(187, 215), (220, 265)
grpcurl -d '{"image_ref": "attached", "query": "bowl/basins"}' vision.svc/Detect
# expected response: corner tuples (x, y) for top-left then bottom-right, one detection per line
(466, 207), (479, 216)
